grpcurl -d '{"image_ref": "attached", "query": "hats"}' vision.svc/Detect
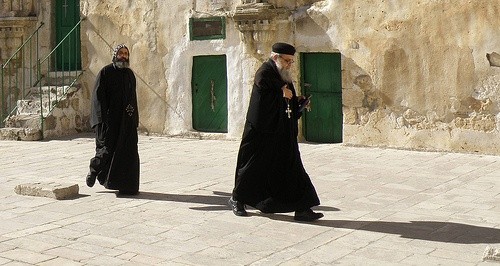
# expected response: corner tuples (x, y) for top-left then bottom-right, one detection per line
(271, 42), (295, 56)
(113, 44), (129, 58)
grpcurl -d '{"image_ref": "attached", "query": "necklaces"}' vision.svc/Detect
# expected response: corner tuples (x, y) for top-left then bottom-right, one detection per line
(282, 82), (292, 119)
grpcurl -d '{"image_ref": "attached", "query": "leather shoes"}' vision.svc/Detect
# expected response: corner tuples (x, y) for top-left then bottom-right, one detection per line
(229, 197), (247, 216)
(295, 209), (324, 221)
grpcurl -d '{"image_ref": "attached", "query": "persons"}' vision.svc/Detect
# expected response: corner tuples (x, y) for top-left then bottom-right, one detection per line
(86, 44), (140, 194)
(227, 42), (323, 221)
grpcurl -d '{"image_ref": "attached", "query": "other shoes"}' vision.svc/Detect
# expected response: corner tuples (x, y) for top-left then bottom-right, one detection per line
(119, 190), (139, 196)
(85, 165), (96, 188)
